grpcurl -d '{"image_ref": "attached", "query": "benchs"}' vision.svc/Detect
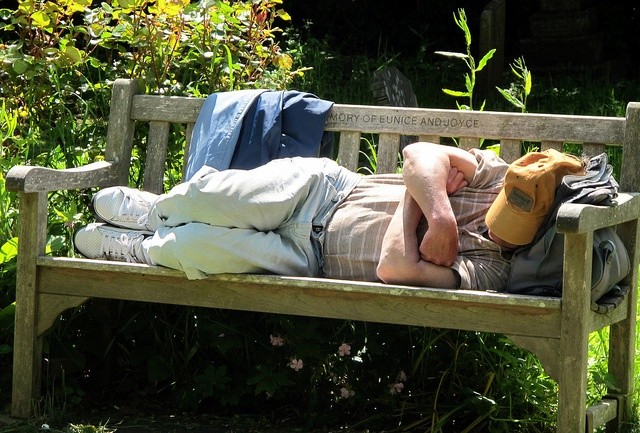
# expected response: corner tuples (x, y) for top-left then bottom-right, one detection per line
(5, 77), (640, 433)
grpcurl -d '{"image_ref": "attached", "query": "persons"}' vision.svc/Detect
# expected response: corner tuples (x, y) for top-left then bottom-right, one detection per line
(74, 142), (589, 289)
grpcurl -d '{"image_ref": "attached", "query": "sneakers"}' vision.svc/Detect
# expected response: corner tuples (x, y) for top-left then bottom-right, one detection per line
(94, 186), (163, 230)
(73, 223), (154, 264)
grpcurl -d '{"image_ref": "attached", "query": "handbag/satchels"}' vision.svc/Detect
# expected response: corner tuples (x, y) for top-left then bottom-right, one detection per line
(510, 188), (630, 304)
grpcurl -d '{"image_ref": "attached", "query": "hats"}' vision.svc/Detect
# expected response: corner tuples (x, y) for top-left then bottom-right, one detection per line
(485, 150), (583, 247)
